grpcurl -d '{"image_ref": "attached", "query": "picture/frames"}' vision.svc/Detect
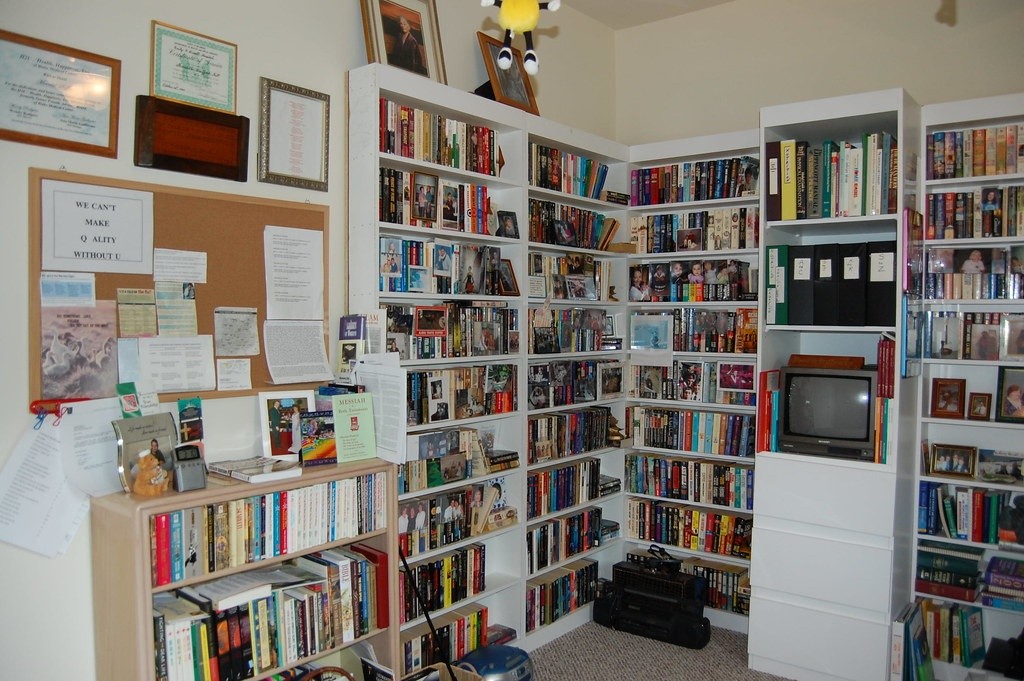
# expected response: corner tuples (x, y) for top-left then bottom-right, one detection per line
(553, 217), (577, 246)
(995, 366), (1024, 423)
(931, 377), (966, 418)
(931, 443), (976, 481)
(968, 392), (991, 419)
(565, 274), (598, 299)
(716, 360), (756, 393)
(495, 258), (519, 295)
(361, 0), (449, 85)
(496, 211), (519, 237)
(411, 171), (440, 221)
(258, 74), (330, 190)
(258, 390), (317, 455)
(477, 31), (540, 116)
(997, 313), (1024, 363)
(595, 362), (624, 404)
(1, 30), (122, 159)
(149, 20), (239, 114)
(598, 314), (615, 336)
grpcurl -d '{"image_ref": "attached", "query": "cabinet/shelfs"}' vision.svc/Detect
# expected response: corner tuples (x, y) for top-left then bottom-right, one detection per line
(911, 93), (1024, 680)
(344, 65), (632, 681)
(615, 126), (764, 638)
(745, 86), (913, 681)
(90, 456), (399, 681)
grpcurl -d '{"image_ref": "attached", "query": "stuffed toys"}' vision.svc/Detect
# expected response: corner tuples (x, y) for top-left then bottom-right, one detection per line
(133, 449), (169, 497)
(481, 0), (561, 75)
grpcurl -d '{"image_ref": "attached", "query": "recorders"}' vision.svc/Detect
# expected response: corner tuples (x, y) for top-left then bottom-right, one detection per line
(459, 644), (533, 681)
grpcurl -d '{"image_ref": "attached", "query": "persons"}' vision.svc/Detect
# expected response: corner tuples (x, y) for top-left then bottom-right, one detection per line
(982, 191), (998, 210)
(269, 401), (281, 447)
(960, 250), (985, 274)
(937, 454), (969, 473)
(287, 404), (301, 454)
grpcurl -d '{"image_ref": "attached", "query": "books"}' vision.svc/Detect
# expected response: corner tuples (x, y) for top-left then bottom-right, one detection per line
(915, 247), (1024, 300)
(915, 312), (1024, 361)
(757, 369), (781, 453)
(149, 472), (389, 681)
(926, 186), (1024, 240)
(926, 123), (1024, 180)
(765, 130), (898, 327)
(889, 480), (1024, 681)
(209, 456), (302, 484)
(303, 314), (377, 467)
(379, 96), (759, 676)
(874, 331), (895, 465)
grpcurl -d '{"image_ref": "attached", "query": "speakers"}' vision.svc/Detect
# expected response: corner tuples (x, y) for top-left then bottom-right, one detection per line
(614, 561), (706, 620)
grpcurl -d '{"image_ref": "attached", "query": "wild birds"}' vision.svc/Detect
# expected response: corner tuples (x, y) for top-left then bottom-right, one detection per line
(42, 331), (116, 377)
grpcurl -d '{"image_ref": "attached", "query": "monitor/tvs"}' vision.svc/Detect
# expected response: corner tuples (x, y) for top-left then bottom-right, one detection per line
(777, 366), (878, 461)
